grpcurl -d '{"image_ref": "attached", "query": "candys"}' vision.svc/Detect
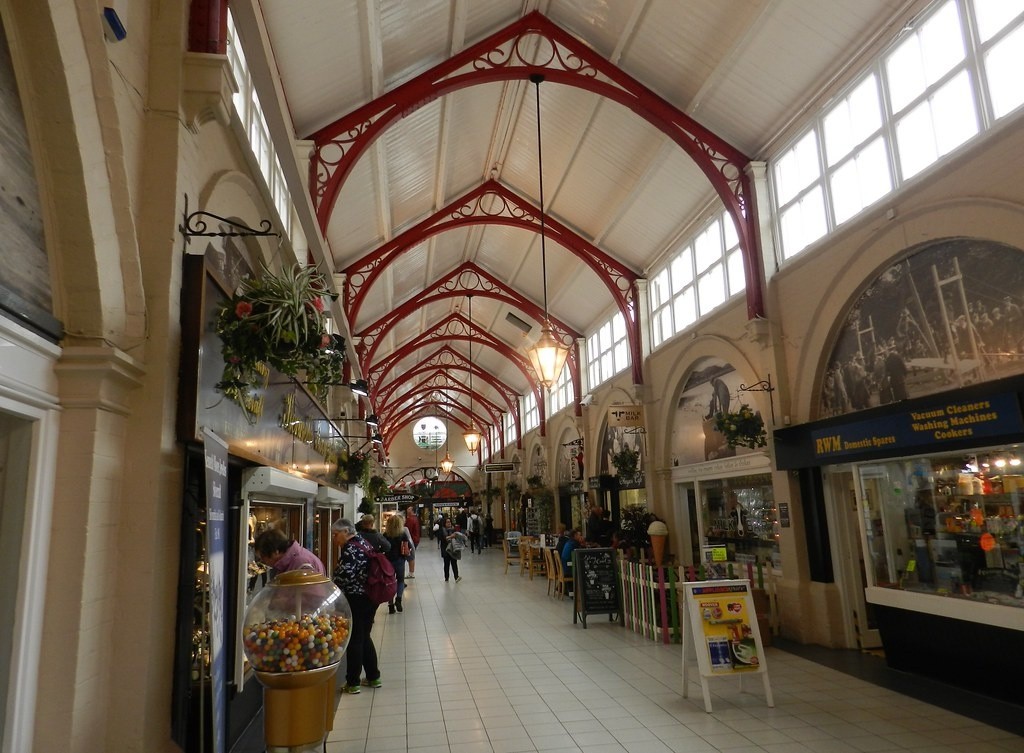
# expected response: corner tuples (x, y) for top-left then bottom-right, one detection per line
(242, 611), (351, 672)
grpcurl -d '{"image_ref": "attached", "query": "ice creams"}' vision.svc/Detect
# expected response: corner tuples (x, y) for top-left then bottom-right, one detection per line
(647, 521), (669, 568)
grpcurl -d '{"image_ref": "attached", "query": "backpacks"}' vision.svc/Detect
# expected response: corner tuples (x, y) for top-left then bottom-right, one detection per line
(470, 515), (480, 532)
(348, 539), (397, 604)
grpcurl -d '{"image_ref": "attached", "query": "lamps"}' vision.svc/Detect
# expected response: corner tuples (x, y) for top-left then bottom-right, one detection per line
(461, 293), (481, 456)
(440, 368), (455, 475)
(982, 456), (990, 468)
(302, 379), (368, 397)
(325, 333), (345, 355)
(526, 73), (571, 393)
(332, 415), (378, 426)
(344, 434), (382, 444)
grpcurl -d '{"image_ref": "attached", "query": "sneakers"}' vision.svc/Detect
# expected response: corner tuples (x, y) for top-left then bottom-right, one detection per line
(361, 676), (382, 688)
(344, 683), (361, 694)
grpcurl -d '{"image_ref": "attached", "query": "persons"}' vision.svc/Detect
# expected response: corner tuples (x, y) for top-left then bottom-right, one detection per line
(611, 531), (633, 560)
(707, 376), (731, 414)
(404, 506), (420, 579)
(438, 518), (462, 583)
(247, 571), (267, 593)
(455, 509), (494, 554)
(906, 295), (1024, 363)
(253, 529), (326, 611)
(584, 507), (614, 546)
(821, 337), (908, 416)
(555, 528), (588, 597)
(382, 514), (412, 614)
(330, 518), (382, 693)
(355, 515), (391, 554)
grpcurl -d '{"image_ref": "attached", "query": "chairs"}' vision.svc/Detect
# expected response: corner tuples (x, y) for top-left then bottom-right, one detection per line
(502, 536), (574, 601)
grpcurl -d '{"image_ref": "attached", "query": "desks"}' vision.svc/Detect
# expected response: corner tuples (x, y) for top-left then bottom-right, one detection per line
(530, 542), (557, 576)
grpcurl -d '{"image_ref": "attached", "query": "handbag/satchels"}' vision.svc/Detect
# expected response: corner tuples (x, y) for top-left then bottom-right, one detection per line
(433, 524), (439, 531)
(400, 540), (409, 555)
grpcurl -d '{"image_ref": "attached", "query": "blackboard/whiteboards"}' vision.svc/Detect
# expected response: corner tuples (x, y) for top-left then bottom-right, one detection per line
(572, 548), (622, 614)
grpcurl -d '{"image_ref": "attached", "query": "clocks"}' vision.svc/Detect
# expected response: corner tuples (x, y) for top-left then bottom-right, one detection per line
(425, 468), (436, 479)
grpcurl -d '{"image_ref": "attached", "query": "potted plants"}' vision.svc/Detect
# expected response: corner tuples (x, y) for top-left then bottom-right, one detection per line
(357, 497), (377, 518)
(368, 476), (390, 498)
(505, 481), (521, 500)
(610, 445), (640, 477)
(618, 503), (666, 560)
(525, 474), (543, 498)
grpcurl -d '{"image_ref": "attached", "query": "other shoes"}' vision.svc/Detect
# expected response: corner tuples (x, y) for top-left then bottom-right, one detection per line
(388, 604), (396, 614)
(456, 576), (461, 583)
(404, 575), (415, 579)
(395, 600), (403, 612)
(445, 578), (449, 583)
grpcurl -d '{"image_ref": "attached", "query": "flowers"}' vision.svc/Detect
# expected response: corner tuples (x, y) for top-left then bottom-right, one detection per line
(205, 256), (348, 426)
(712, 404), (767, 449)
(347, 450), (371, 487)
